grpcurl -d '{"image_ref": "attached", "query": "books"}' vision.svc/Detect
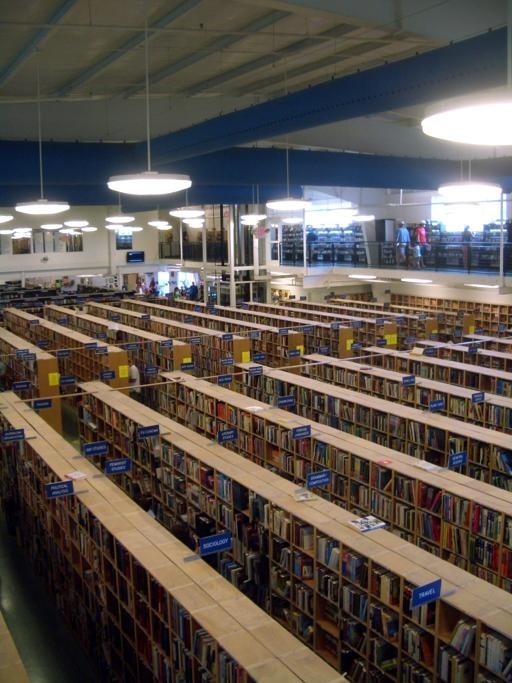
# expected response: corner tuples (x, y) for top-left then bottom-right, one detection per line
(1, 293), (511, 683)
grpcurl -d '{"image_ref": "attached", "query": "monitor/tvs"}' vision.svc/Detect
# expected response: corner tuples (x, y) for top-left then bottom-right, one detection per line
(127, 251), (144, 262)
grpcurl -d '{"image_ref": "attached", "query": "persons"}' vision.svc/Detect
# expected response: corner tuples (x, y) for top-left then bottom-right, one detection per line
(409, 242), (422, 269)
(395, 221), (410, 265)
(416, 220), (426, 268)
(463, 225), (475, 245)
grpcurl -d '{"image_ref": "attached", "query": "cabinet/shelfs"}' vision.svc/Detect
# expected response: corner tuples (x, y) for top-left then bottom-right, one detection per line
(0, 282), (512, 683)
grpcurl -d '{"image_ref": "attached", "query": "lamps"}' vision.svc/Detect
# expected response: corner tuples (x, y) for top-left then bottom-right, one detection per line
(0, 0), (512, 240)
